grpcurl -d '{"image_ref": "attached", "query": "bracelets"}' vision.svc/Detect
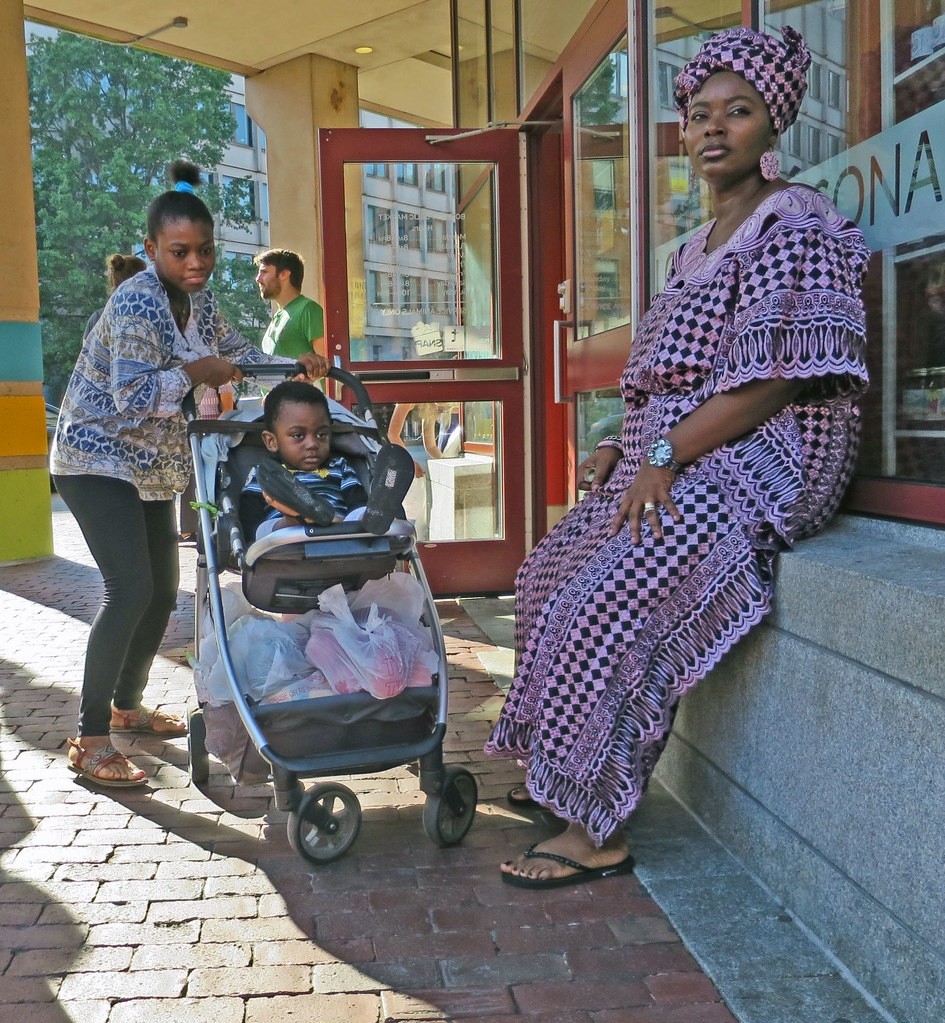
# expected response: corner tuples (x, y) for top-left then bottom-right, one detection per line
(595, 435), (623, 453)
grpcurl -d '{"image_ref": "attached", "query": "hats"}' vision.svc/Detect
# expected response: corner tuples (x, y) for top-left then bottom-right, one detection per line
(673, 25), (814, 179)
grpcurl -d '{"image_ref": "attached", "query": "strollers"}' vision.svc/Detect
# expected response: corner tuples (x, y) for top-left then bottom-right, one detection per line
(177, 353), (483, 870)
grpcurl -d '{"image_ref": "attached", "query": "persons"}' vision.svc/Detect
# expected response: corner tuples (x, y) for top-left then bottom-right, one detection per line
(193, 381), (237, 505)
(50, 158), (330, 788)
(239, 380), (418, 540)
(352, 402), (444, 520)
(492, 22), (868, 893)
(83, 252), (147, 342)
(251, 248), (325, 359)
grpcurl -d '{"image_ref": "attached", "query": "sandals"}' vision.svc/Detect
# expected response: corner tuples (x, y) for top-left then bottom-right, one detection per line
(363, 444), (414, 535)
(68, 738), (148, 786)
(108, 704), (189, 735)
(256, 461), (334, 528)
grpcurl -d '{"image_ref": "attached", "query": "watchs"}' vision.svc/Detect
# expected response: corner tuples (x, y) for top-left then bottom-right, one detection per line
(647, 438), (683, 475)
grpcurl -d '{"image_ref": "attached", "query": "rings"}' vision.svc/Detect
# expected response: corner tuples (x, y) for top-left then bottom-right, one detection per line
(644, 502), (655, 514)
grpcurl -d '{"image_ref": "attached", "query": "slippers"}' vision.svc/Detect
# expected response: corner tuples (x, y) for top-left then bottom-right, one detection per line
(502, 843), (635, 888)
(508, 786), (543, 805)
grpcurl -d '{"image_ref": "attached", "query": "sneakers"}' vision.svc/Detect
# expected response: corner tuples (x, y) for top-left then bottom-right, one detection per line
(178, 533), (198, 547)
